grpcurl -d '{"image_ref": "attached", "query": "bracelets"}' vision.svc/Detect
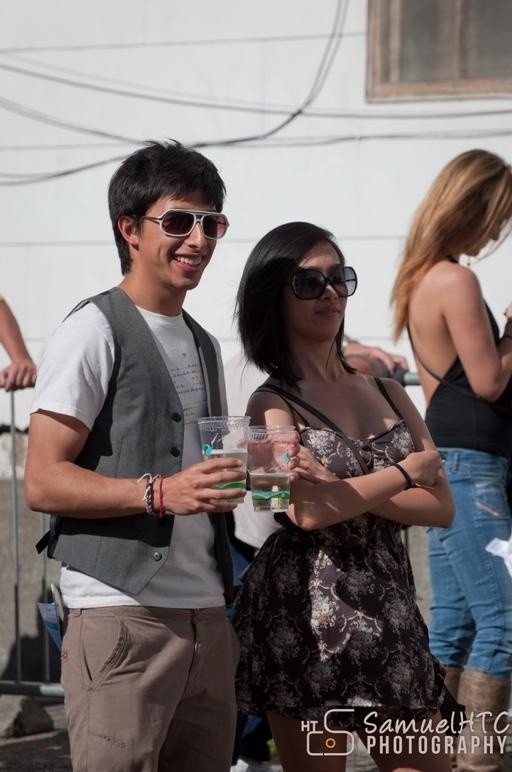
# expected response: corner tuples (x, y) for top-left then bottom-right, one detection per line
(390, 463), (411, 490)
(145, 474), (158, 516)
(502, 334), (512, 338)
(160, 476), (166, 519)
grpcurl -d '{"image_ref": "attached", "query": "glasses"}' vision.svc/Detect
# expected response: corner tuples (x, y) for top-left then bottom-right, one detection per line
(133, 208), (229, 240)
(292, 267), (356, 300)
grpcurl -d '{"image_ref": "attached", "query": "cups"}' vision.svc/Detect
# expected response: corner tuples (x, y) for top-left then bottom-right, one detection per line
(194, 417), (298, 513)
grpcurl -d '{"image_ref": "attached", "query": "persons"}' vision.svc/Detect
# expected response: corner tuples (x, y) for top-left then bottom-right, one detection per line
(390, 149), (512, 772)
(230, 222), (456, 772)
(23, 138), (301, 772)
(0, 294), (37, 392)
